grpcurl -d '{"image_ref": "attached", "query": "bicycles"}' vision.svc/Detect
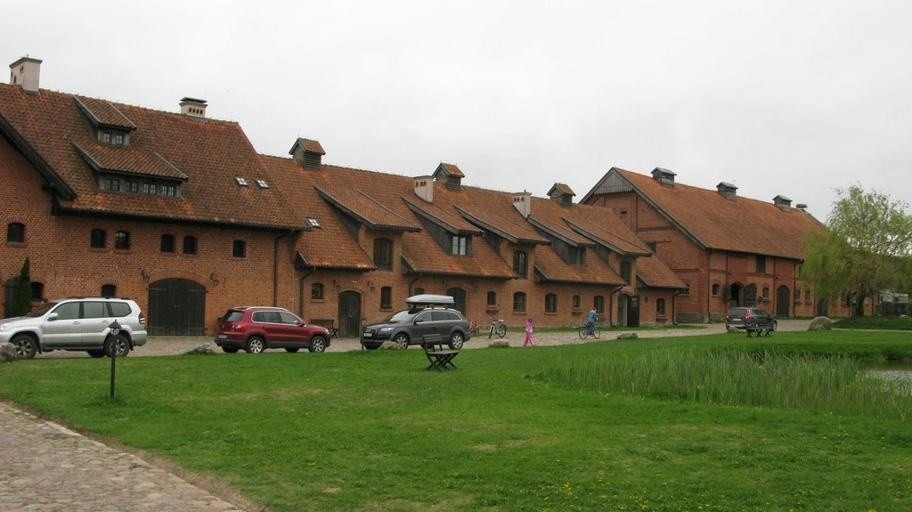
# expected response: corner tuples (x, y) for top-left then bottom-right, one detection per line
(488, 319), (506, 340)
(467, 319), (480, 334)
(577, 324), (600, 340)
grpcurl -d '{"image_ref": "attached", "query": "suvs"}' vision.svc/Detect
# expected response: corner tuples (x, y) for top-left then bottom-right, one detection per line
(725, 307), (777, 331)
(0, 298), (148, 362)
(214, 307), (332, 354)
(359, 294), (471, 351)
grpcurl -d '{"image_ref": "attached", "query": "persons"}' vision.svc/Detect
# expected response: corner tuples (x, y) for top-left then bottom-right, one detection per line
(523, 317), (535, 347)
(587, 306), (598, 332)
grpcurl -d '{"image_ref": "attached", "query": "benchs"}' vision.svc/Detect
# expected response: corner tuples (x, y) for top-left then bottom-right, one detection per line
(423, 334), (459, 371)
(310, 318), (339, 339)
